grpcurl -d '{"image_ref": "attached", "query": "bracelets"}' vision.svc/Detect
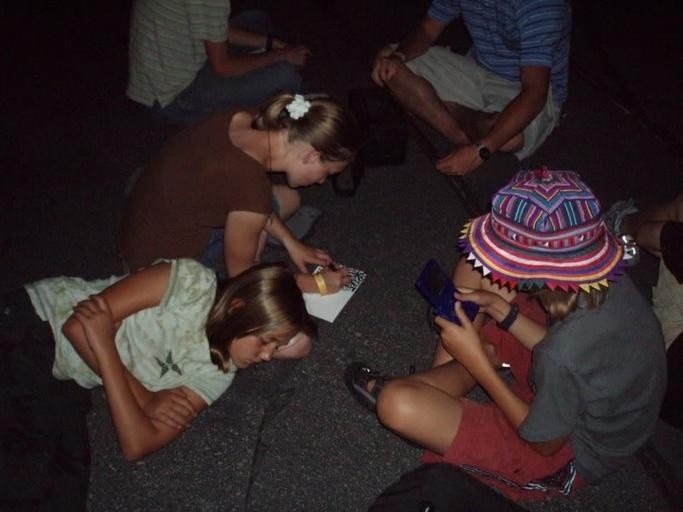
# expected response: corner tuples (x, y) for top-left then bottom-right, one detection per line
(265, 35), (273, 50)
(311, 272), (329, 296)
(494, 302), (520, 333)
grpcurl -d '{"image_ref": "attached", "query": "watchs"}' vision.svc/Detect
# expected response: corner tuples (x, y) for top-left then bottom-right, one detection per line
(469, 138), (494, 164)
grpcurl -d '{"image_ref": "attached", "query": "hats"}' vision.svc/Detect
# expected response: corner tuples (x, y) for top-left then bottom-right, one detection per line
(457, 163), (630, 295)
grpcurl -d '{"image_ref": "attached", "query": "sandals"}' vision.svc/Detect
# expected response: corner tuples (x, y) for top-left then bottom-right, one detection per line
(346, 364), (388, 410)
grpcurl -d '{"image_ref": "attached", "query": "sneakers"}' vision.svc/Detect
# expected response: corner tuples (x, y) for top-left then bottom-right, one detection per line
(604, 198), (641, 232)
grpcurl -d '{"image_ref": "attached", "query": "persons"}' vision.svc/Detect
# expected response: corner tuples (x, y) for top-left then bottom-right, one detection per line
(368, 1), (572, 178)
(106, 89), (360, 363)
(0, 258), (309, 512)
(121, 1), (317, 133)
(597, 199), (681, 433)
(342, 166), (670, 500)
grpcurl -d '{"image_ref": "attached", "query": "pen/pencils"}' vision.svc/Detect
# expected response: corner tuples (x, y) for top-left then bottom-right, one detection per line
(327, 261), (337, 273)
